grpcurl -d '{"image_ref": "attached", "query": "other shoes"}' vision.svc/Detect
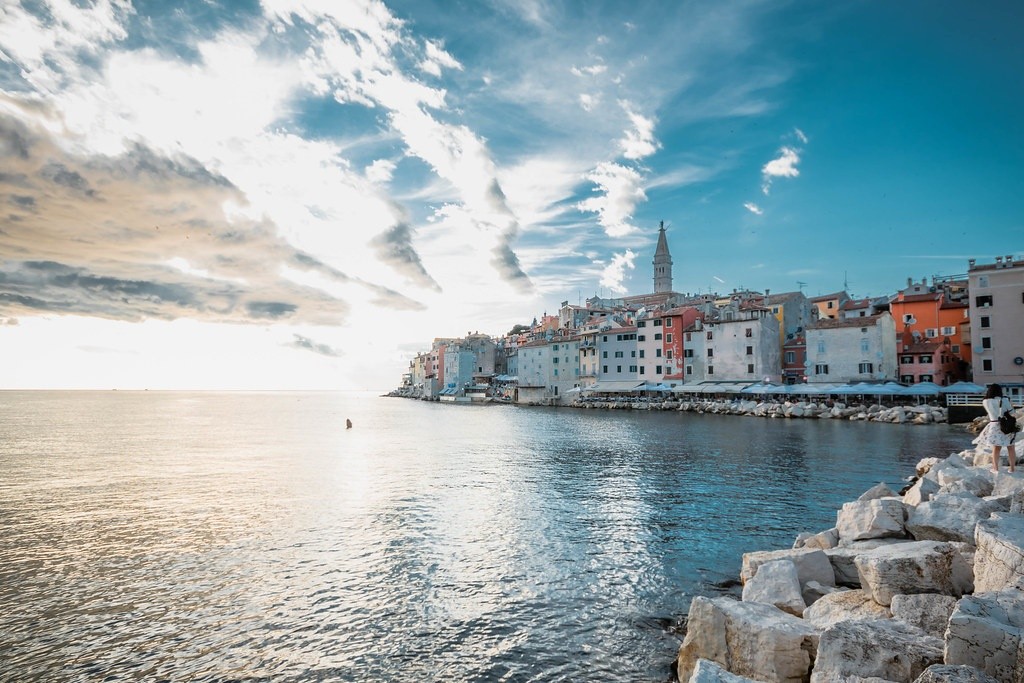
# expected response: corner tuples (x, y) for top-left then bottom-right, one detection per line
(990, 469), (998, 474)
(1007, 470), (1014, 474)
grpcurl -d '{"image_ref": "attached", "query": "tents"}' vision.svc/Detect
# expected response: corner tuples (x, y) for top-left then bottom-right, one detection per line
(634, 385), (672, 397)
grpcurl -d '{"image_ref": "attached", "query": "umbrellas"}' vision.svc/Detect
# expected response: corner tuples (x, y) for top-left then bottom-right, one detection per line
(566, 387), (580, 403)
(769, 382), (987, 405)
(742, 382), (776, 398)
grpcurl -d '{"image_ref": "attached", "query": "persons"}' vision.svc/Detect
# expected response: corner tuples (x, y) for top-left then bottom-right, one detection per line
(972, 384), (1024, 475)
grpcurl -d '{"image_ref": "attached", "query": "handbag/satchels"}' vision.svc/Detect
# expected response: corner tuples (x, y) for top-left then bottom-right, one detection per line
(998, 411), (1016, 434)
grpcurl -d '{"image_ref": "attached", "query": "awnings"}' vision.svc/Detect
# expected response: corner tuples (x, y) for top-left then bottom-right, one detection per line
(439, 389), (458, 394)
(497, 376), (518, 380)
(465, 386), (489, 389)
(583, 380), (645, 393)
(672, 386), (747, 396)
